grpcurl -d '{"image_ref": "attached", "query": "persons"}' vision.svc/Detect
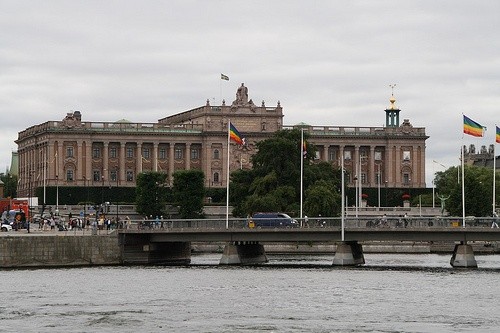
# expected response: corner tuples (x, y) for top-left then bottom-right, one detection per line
(490, 212), (499, 228)
(139, 215), (166, 231)
(304, 215), (310, 228)
(436, 192), (451, 216)
(14, 211), (132, 232)
(317, 214), (324, 227)
(382, 212), (409, 228)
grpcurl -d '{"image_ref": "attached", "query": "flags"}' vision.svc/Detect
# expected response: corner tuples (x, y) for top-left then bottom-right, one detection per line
(302, 131), (308, 158)
(463, 115), (487, 137)
(496, 126), (500, 143)
(221, 74), (229, 81)
(229, 121), (243, 145)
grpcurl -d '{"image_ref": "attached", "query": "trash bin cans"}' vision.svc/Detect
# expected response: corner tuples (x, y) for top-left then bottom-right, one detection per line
(92, 227), (97, 235)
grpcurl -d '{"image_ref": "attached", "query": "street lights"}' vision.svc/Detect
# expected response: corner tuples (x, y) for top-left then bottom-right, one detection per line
(354, 174), (359, 216)
(100, 175), (105, 206)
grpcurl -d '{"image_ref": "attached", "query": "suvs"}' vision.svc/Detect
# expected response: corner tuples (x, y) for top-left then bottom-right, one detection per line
(253, 212), (299, 228)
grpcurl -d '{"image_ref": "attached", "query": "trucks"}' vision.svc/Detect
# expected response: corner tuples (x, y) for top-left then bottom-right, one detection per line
(0, 197), (29, 229)
